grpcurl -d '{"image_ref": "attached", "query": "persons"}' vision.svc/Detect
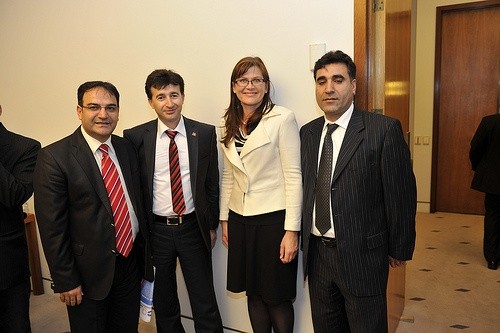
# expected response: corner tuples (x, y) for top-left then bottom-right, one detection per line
(217, 57), (303, 333)
(34, 81), (155, 333)
(0, 105), (41, 333)
(469, 114), (500, 271)
(124, 70), (223, 333)
(300, 50), (417, 333)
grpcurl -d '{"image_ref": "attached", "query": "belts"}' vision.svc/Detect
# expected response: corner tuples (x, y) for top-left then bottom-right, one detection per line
(151, 210), (197, 224)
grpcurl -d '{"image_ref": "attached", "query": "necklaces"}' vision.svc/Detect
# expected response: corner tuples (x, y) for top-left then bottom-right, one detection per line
(241, 121), (247, 125)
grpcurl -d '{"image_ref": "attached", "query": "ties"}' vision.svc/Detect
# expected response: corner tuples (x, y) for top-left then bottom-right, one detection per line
(315, 123), (339, 235)
(98, 143), (133, 257)
(165, 130), (186, 215)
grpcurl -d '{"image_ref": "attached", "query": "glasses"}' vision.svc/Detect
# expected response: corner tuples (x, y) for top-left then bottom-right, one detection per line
(82, 104), (119, 111)
(234, 77), (265, 85)
